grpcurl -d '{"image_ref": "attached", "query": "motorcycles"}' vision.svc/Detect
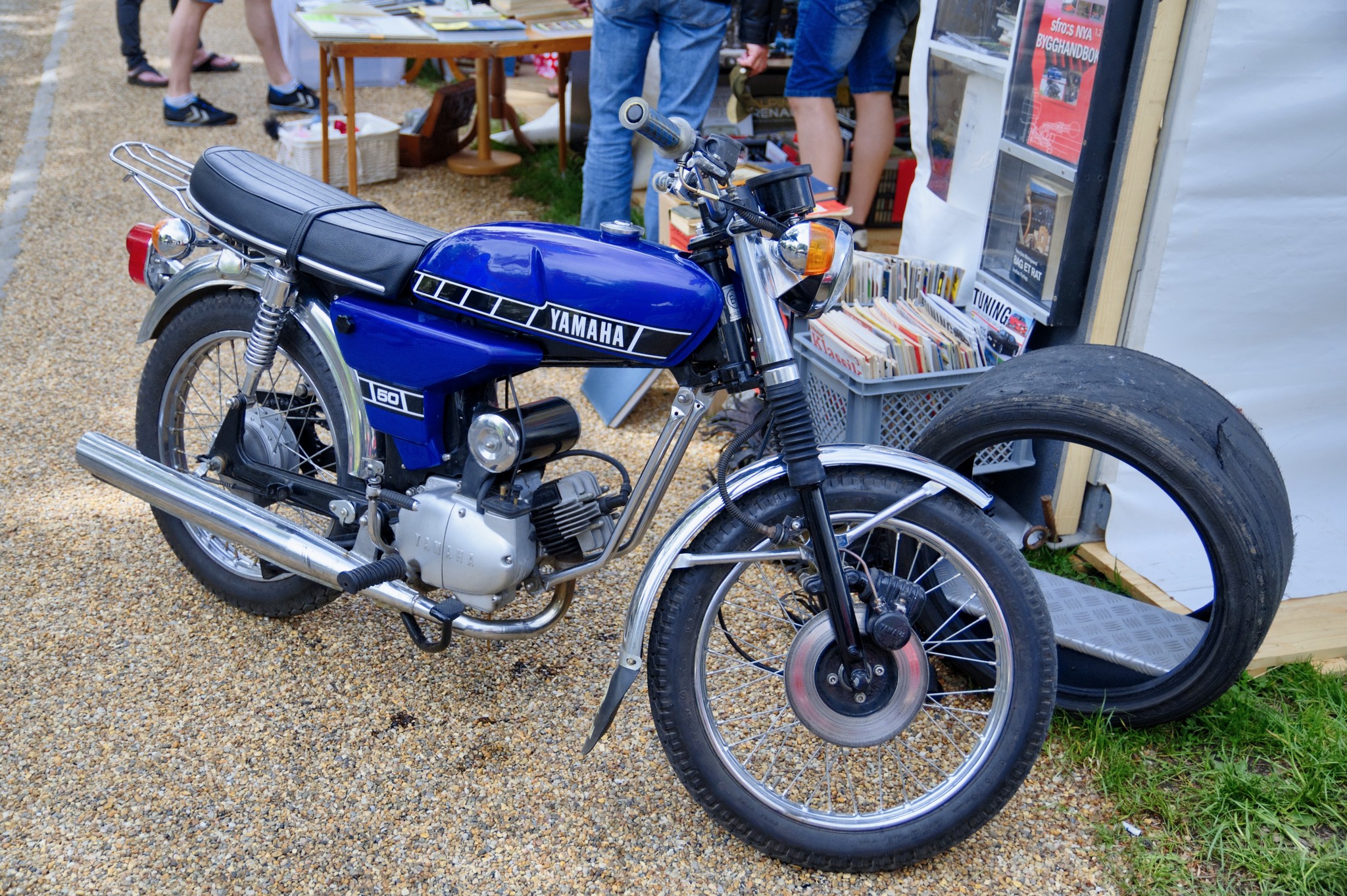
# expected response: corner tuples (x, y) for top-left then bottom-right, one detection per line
(71, 95), (1059, 875)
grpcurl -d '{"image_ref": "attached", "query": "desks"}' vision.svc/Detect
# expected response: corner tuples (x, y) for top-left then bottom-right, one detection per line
(298, 12), (596, 202)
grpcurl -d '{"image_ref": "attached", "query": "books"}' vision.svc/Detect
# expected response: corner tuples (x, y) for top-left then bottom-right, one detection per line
(290, 0), (594, 40)
(835, 107), (856, 160)
(765, 133), (799, 162)
(808, 250), (1035, 448)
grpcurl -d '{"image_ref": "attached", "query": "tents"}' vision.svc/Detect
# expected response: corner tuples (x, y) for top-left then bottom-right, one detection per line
(668, 163), (853, 254)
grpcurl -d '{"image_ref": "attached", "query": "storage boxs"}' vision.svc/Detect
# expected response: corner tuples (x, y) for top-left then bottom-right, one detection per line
(282, 110), (402, 191)
(790, 310), (1040, 482)
(728, 109), (927, 230)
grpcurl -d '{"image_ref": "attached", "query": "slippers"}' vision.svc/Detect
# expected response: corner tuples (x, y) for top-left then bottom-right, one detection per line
(192, 52), (238, 72)
(128, 65), (167, 87)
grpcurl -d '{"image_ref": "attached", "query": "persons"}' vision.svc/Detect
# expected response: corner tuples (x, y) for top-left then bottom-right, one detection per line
(531, 52), (559, 97)
(804, 249), (807, 258)
(116, 0), (240, 87)
(164, 0), (337, 126)
(787, 245), (791, 253)
(568, 0), (785, 243)
(784, 0), (920, 253)
(795, 246), (799, 257)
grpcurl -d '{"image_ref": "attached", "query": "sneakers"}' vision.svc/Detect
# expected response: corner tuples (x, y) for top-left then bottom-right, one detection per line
(163, 94), (236, 126)
(267, 81), (335, 115)
